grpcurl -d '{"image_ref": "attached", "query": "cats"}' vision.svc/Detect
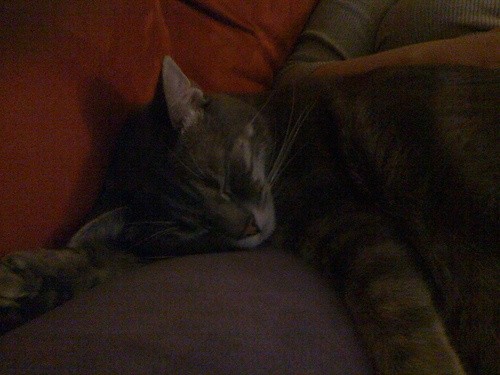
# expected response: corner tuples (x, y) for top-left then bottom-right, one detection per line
(1, 54), (500, 375)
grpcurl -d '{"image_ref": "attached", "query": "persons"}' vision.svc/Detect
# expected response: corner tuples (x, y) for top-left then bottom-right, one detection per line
(280, 1), (499, 82)
